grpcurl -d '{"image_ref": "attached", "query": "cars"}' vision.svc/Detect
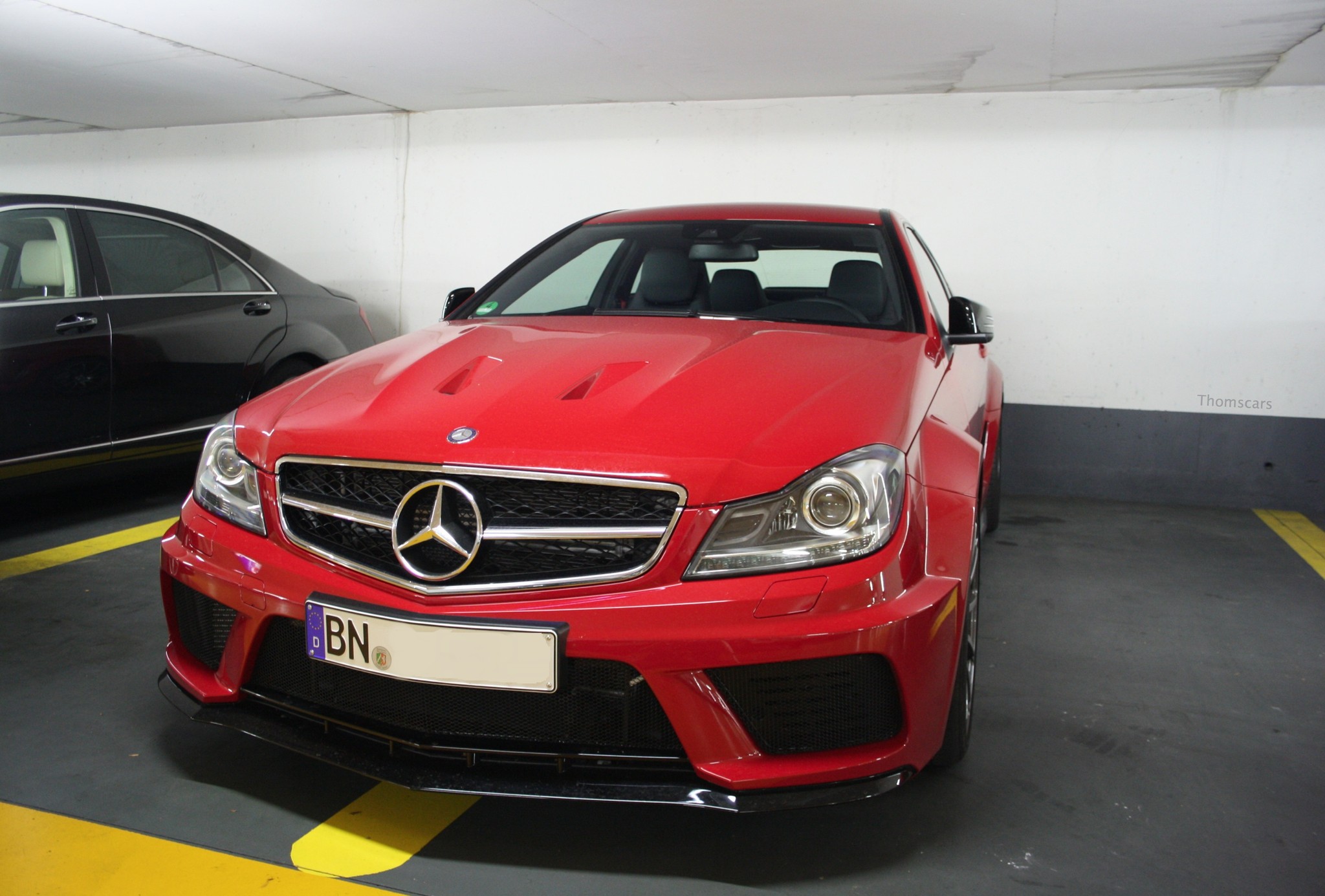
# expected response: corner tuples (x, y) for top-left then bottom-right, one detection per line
(160, 202), (1006, 815)
(1, 193), (379, 500)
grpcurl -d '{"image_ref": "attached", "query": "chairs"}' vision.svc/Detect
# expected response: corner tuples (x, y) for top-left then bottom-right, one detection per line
(20, 239), (61, 302)
(827, 259), (887, 321)
(710, 269), (765, 317)
(630, 253), (708, 312)
